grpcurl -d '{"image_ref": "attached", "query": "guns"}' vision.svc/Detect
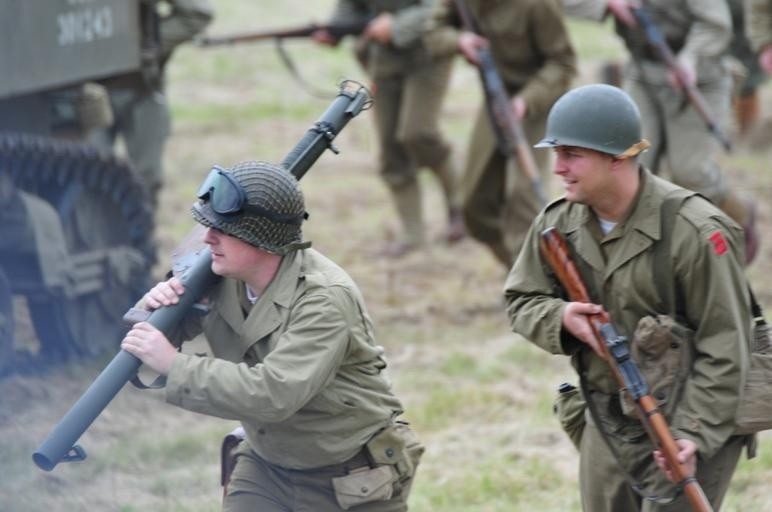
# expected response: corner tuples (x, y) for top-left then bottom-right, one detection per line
(630, 0), (731, 152)
(195, 14), (370, 50)
(454, 0), (547, 208)
(538, 226), (714, 512)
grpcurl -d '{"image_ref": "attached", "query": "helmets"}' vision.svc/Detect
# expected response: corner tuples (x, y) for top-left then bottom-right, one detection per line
(189, 161), (305, 255)
(534, 83), (647, 156)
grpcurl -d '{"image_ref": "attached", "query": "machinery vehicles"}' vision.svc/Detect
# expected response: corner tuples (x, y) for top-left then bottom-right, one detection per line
(0, 0), (214, 380)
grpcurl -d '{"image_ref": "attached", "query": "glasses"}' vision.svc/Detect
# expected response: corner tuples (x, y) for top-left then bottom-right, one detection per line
(198, 165), (302, 225)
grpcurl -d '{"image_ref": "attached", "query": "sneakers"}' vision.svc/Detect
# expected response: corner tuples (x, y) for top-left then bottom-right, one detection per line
(448, 207), (466, 240)
(743, 196), (758, 263)
(383, 243), (412, 260)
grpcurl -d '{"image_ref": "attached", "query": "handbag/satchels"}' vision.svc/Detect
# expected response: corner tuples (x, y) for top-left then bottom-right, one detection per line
(620, 315), (772, 435)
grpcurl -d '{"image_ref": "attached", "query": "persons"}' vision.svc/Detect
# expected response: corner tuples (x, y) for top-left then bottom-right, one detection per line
(425, 0), (579, 267)
(503, 83), (758, 512)
(561, 0), (758, 266)
(313, 0), (467, 252)
(120, 161), (425, 512)
(722, 0), (772, 139)
